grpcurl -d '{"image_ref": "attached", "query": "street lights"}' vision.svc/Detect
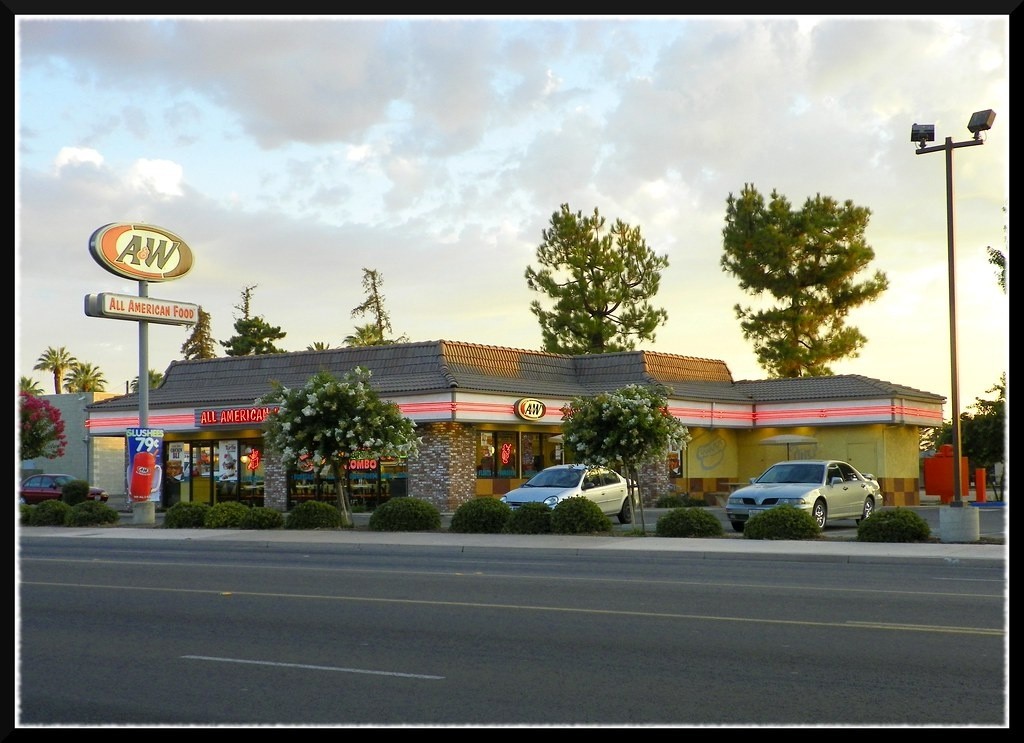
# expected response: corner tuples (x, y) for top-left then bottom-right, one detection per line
(910, 109), (999, 543)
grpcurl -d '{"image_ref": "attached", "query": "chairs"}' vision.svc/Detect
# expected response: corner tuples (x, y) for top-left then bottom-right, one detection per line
(477, 457), (493, 476)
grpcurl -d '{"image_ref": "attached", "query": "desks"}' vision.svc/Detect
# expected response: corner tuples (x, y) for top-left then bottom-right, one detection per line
(719, 483), (750, 493)
(245, 494), (390, 508)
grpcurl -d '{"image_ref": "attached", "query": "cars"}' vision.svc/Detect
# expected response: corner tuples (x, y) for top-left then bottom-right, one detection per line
(727, 459), (885, 533)
(500, 463), (641, 526)
(21, 473), (109, 508)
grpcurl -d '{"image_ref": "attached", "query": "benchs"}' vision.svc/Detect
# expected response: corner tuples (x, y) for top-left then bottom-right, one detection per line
(709, 492), (730, 508)
